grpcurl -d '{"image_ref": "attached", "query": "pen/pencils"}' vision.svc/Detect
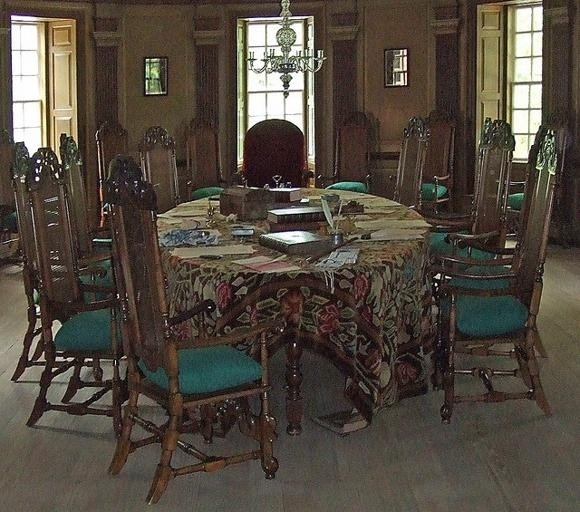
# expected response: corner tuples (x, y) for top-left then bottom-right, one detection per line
(337, 201), (343, 230)
(308, 237), (359, 264)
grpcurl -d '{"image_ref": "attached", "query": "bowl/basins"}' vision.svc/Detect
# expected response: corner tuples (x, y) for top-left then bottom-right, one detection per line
(321, 194), (339, 202)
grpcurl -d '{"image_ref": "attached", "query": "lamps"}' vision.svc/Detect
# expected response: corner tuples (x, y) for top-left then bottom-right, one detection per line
(245, 0), (328, 98)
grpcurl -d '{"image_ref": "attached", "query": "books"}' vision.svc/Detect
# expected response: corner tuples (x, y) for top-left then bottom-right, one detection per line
(260, 232), (330, 254)
(260, 188), (328, 232)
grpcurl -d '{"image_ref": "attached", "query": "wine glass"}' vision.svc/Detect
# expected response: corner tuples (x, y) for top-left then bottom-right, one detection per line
(272, 175), (282, 189)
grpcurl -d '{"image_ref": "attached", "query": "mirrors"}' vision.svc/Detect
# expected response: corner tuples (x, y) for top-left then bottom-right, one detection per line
(143, 56), (168, 96)
(384, 48), (409, 88)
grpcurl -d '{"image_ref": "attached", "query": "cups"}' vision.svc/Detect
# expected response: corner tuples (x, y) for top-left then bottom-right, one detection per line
(264, 183), (269, 191)
(279, 182), (292, 189)
(332, 232), (344, 245)
